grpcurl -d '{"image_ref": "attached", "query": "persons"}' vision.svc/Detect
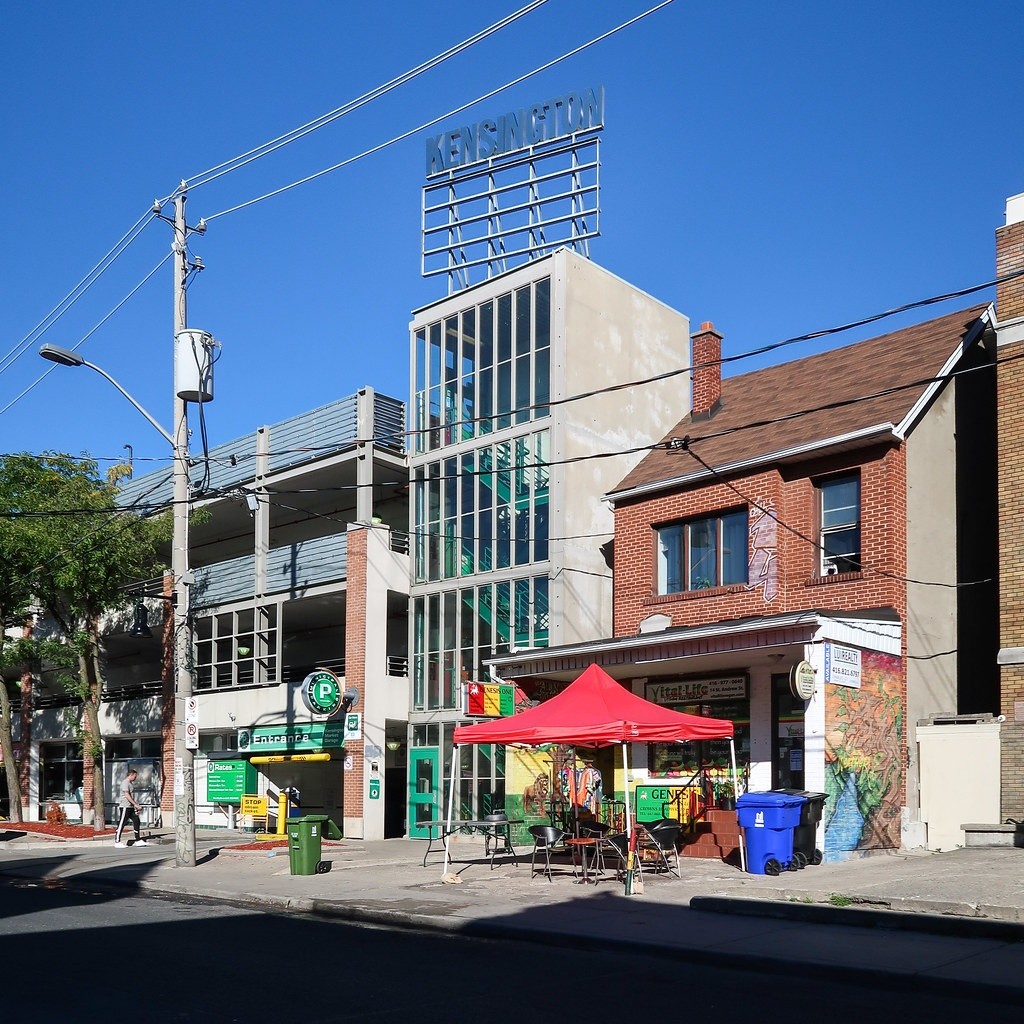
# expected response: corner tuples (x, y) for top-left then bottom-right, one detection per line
(115, 770), (149, 849)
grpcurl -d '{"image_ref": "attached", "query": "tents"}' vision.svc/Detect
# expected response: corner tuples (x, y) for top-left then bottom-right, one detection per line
(439, 663), (745, 873)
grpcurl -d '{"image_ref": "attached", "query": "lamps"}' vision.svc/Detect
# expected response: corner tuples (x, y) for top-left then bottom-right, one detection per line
(312, 749), (324, 754)
(238, 643), (250, 655)
(385, 737), (401, 750)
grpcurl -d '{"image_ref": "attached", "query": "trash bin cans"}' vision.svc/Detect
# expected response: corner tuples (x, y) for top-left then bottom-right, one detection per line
(769, 788), (830, 869)
(286, 815), (343, 876)
(735, 791), (807, 876)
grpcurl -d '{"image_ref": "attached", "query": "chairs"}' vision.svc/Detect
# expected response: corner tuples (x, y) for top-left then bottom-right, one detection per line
(578, 821), (620, 872)
(528, 825), (578, 882)
(635, 825), (681, 879)
(485, 813), (511, 857)
(594, 825), (644, 884)
(644, 819), (665, 830)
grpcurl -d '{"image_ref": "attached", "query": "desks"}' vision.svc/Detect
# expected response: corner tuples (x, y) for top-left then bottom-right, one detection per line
(563, 837), (600, 884)
(416, 820), (524, 870)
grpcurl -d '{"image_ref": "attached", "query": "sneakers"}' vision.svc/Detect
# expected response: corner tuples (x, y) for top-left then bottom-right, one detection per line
(115, 842), (126, 849)
(135, 840), (147, 846)
